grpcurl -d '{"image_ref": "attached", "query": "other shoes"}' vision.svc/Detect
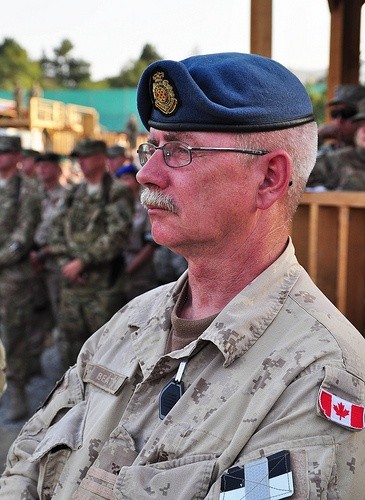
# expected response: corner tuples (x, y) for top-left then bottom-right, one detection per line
(5, 385), (28, 419)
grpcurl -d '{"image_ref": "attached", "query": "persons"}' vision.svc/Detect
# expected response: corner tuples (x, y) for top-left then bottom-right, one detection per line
(0, 84), (365, 426)
(0, 53), (365, 500)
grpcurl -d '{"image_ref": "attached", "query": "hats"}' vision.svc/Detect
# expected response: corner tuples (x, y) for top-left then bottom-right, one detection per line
(34, 153), (59, 164)
(352, 99), (365, 120)
(107, 147), (125, 158)
(117, 165), (136, 177)
(70, 141), (106, 157)
(23, 150), (39, 156)
(136, 52), (314, 131)
(0, 136), (21, 153)
(328, 84), (365, 104)
(318, 125), (342, 140)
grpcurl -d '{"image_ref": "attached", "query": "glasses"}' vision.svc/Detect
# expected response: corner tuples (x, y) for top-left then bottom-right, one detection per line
(136, 141), (293, 186)
(331, 108), (357, 120)
